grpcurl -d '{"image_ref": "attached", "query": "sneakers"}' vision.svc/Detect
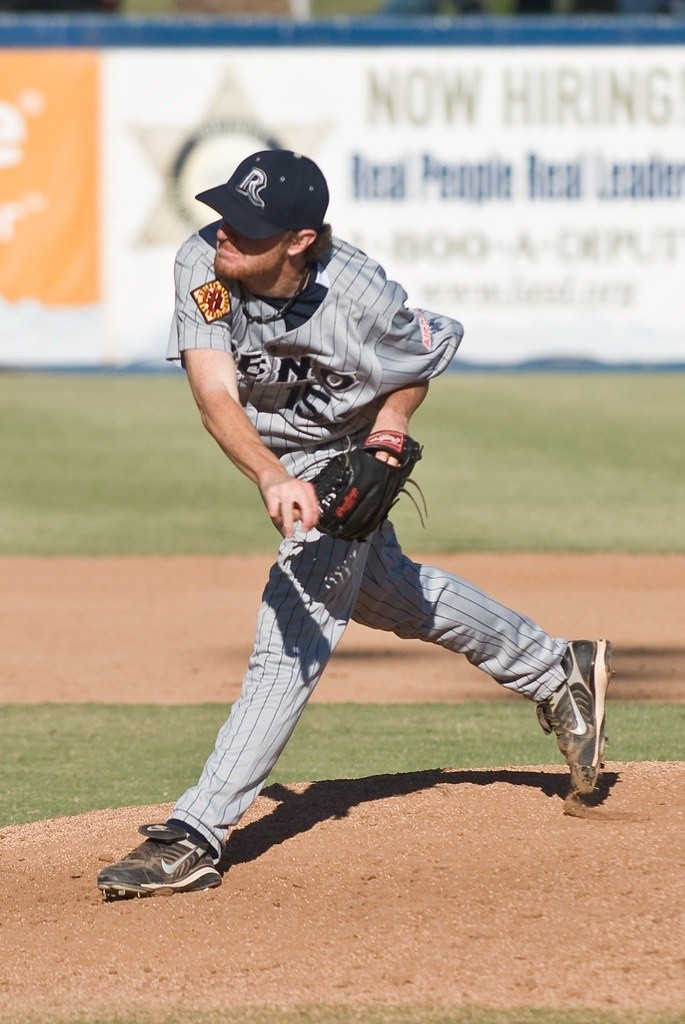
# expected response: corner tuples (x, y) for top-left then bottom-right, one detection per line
(537, 638), (612, 794)
(98, 823), (222, 893)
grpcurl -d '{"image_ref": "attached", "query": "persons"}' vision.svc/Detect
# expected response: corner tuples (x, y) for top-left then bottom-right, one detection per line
(98, 149), (613, 904)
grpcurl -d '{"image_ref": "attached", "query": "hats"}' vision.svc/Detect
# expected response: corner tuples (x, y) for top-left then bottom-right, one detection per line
(194, 149), (329, 240)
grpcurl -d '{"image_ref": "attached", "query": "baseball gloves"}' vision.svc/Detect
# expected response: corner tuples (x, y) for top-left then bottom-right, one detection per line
(306, 430), (428, 544)
